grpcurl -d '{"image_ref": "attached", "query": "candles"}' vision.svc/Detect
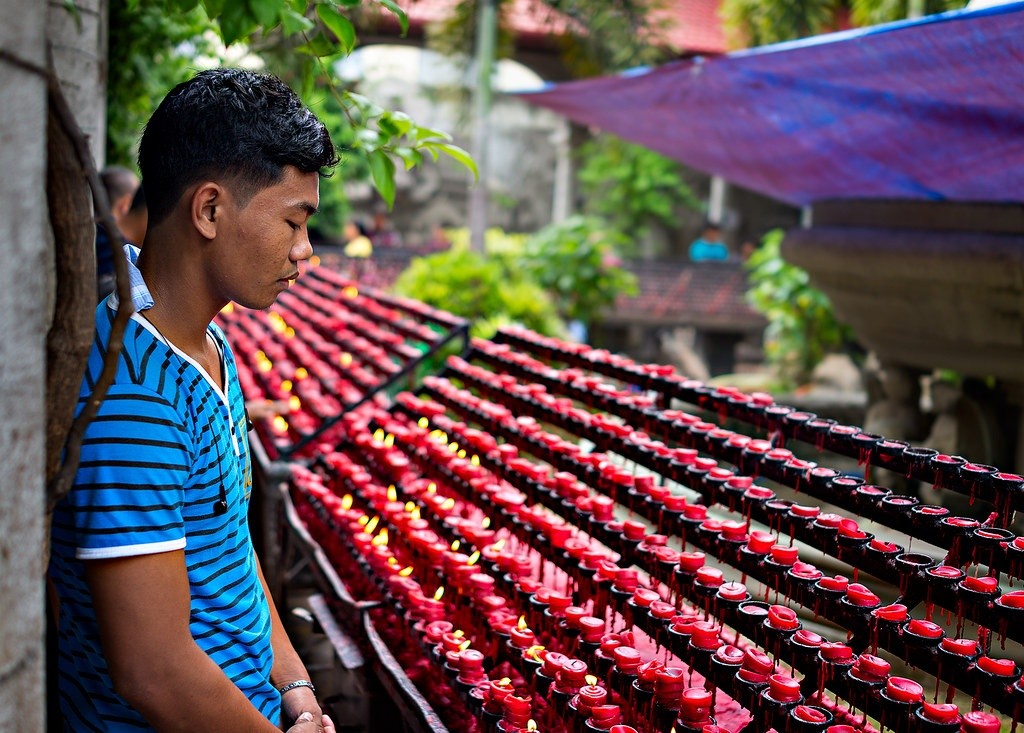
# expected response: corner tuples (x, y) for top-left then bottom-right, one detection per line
(212, 259), (1024, 733)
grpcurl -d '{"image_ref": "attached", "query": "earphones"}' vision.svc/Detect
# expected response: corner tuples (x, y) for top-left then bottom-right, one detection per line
(245, 407), (254, 432)
(214, 484), (228, 514)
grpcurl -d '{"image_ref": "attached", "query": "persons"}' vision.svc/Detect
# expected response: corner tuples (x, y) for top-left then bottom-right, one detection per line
(688, 223), (728, 262)
(95, 166), (145, 275)
(56, 67), (337, 733)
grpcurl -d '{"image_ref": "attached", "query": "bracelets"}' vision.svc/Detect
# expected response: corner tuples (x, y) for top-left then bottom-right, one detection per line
(281, 680), (314, 693)
